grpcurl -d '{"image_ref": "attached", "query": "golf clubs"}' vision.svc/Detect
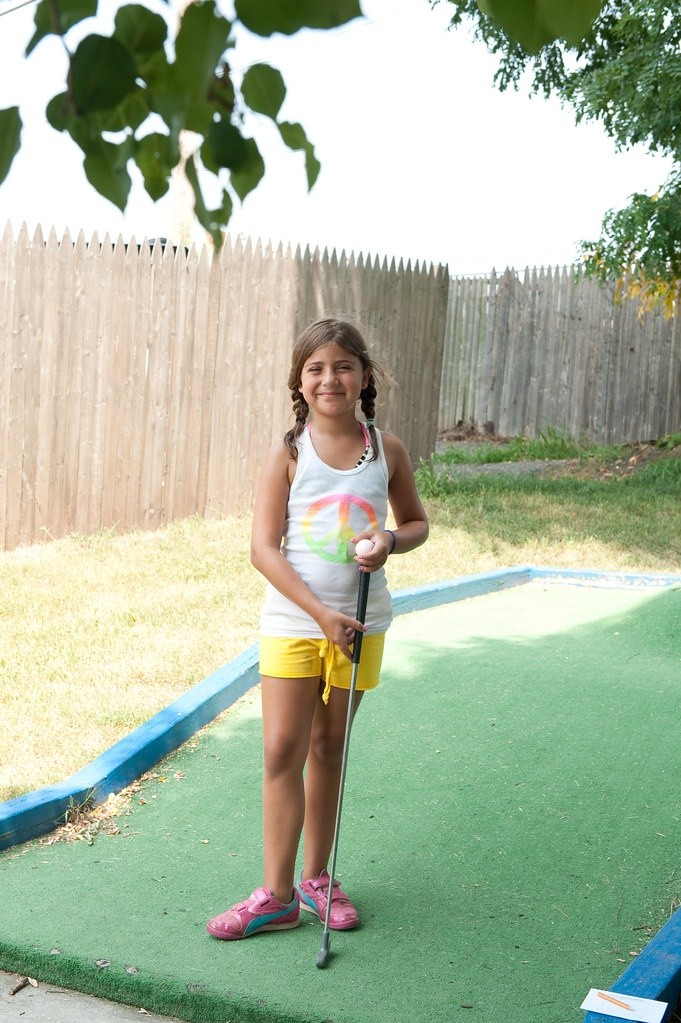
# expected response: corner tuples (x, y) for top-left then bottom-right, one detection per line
(318, 564), (370, 967)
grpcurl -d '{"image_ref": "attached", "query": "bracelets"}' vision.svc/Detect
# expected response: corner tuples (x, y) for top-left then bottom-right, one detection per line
(381, 529), (396, 556)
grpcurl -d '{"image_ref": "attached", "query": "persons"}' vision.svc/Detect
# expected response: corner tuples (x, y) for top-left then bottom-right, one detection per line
(207, 314), (430, 942)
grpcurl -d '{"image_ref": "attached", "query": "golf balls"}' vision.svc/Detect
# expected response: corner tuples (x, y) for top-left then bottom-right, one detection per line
(354, 539), (374, 556)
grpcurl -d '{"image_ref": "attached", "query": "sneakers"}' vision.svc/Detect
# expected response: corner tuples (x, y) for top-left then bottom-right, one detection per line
(206, 888), (302, 941)
(298, 869), (358, 930)
